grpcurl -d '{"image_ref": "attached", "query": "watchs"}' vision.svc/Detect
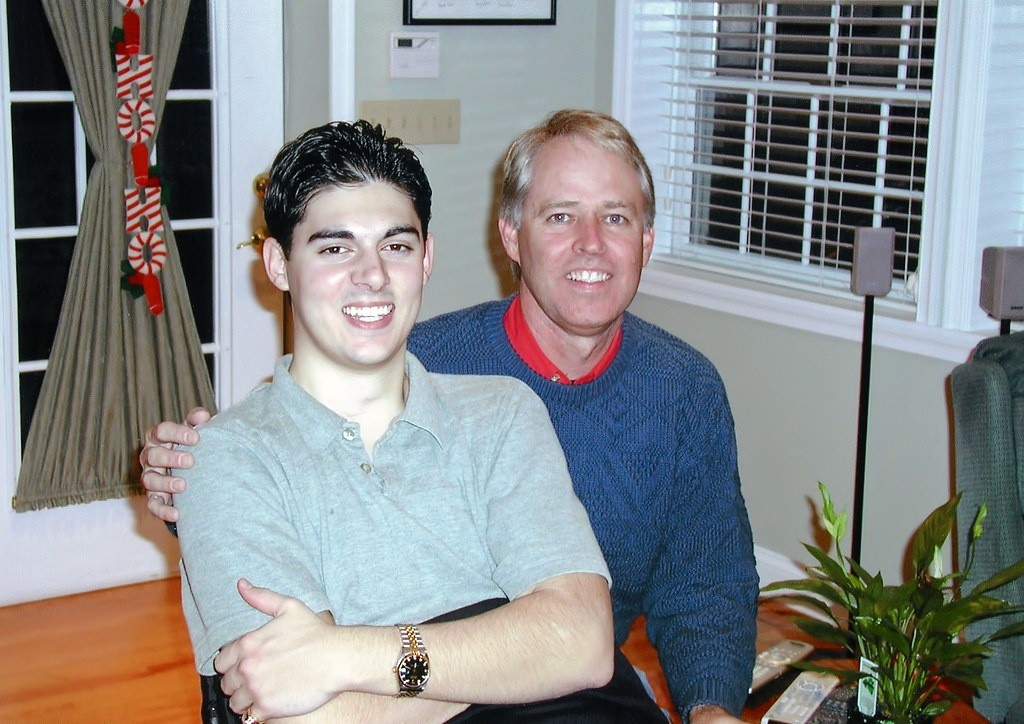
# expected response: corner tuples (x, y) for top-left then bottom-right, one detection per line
(395, 624), (431, 698)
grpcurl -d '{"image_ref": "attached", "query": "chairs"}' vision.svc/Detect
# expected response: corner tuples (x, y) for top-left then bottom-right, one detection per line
(950, 327), (1024, 724)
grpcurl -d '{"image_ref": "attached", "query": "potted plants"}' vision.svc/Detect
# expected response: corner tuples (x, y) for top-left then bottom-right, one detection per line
(752, 489), (1024, 724)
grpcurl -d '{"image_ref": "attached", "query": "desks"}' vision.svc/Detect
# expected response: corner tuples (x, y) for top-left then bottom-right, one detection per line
(0, 575), (995, 724)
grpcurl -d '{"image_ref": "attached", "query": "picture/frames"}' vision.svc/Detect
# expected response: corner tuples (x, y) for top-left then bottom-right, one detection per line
(402, 0), (557, 26)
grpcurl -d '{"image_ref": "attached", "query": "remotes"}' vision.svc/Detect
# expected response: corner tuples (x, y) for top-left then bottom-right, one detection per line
(746, 640), (859, 724)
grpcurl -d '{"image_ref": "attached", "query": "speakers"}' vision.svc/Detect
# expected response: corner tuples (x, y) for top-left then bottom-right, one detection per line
(979, 246), (1024, 320)
(851, 227), (896, 297)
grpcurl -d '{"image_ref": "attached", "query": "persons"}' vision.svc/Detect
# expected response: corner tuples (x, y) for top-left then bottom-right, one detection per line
(140, 109), (759, 724)
(168, 120), (672, 724)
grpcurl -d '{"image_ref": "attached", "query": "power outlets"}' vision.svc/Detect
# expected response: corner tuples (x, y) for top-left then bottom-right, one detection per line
(359, 99), (461, 146)
